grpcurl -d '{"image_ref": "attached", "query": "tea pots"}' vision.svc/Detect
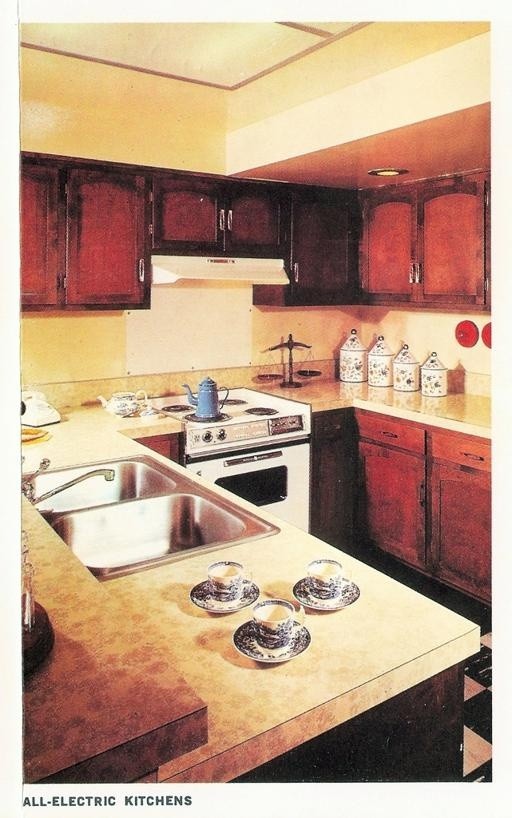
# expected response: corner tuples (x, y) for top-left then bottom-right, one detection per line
(182, 377), (229, 418)
(98, 391), (147, 418)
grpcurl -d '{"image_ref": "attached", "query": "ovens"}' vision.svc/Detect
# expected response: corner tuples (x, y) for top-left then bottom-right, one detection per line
(178, 434), (311, 536)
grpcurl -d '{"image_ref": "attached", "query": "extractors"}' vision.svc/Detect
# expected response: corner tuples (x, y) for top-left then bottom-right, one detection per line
(151, 255), (291, 285)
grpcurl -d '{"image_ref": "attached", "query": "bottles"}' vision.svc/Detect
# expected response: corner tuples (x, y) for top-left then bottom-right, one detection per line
(340, 329), (448, 397)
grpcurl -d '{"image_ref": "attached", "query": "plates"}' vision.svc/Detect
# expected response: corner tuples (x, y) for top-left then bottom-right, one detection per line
(190, 579), (260, 613)
(233, 619), (312, 663)
(292, 577), (360, 610)
(22, 598), (55, 675)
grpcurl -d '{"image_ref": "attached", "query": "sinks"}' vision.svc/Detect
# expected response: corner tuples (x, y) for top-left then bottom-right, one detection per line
(50, 494), (280, 584)
(34, 454), (209, 515)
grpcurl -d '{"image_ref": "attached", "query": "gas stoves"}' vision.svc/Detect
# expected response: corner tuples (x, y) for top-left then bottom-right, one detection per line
(140, 387), (311, 458)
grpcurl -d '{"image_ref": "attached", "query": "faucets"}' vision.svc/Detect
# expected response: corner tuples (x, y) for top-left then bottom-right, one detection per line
(22, 457), (116, 506)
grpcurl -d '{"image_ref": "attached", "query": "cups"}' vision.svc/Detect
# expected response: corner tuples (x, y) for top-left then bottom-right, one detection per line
(306, 559), (352, 601)
(253, 599), (306, 655)
(207, 560), (253, 604)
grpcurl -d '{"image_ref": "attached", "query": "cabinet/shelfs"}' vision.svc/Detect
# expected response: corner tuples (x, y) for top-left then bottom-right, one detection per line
(350, 404), (491, 606)
(354, 166), (491, 319)
(20, 148), (152, 317)
(311, 405), (354, 548)
(131, 434), (179, 465)
(249, 180), (361, 309)
(151, 164), (286, 261)
